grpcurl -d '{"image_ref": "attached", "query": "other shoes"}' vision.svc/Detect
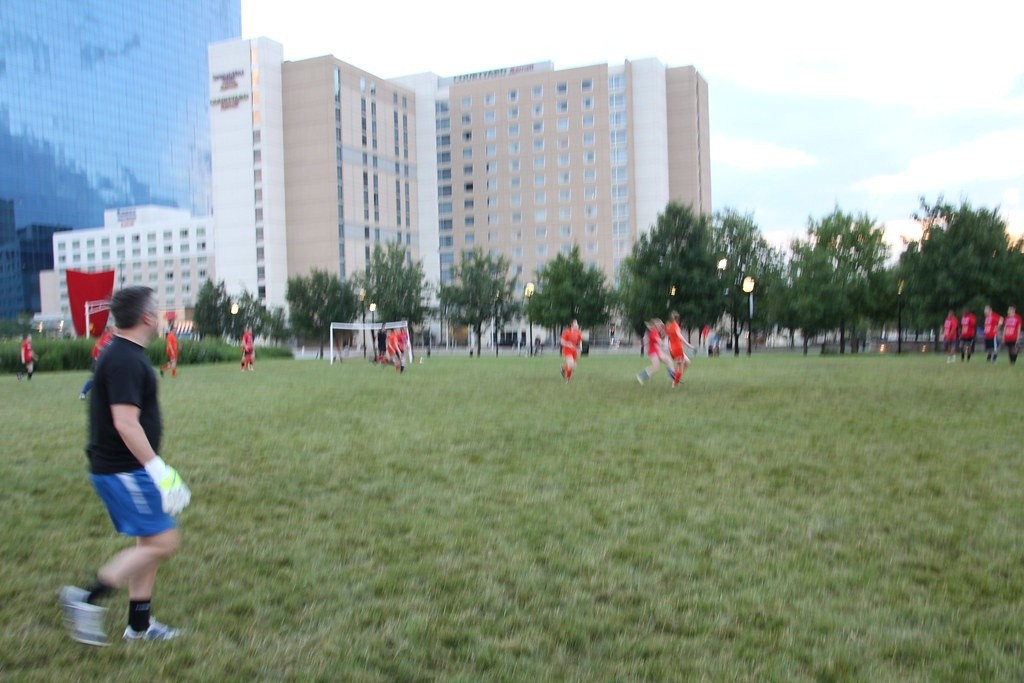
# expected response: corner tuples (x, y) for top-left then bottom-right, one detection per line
(60, 584), (110, 646)
(122, 615), (185, 640)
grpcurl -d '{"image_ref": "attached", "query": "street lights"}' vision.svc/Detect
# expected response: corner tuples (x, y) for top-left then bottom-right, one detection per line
(742, 276), (755, 355)
(524, 282), (535, 355)
(368, 303), (377, 361)
(231, 303), (239, 345)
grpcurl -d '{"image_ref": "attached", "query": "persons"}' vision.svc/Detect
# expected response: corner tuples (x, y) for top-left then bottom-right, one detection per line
(16, 334), (34, 382)
(59, 285), (193, 644)
(959, 307), (977, 361)
(559, 318), (582, 381)
(1003, 305), (1022, 364)
(634, 318), (676, 384)
(376, 323), (407, 372)
(983, 305), (1004, 363)
(160, 329), (178, 377)
(241, 323), (255, 371)
(79, 328), (113, 399)
(665, 310), (697, 388)
(943, 310), (957, 362)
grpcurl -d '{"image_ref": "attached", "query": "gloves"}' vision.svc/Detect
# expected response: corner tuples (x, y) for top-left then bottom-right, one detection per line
(146, 455), (192, 517)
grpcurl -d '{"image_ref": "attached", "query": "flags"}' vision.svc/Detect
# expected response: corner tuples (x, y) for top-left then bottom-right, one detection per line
(65, 268), (114, 335)
